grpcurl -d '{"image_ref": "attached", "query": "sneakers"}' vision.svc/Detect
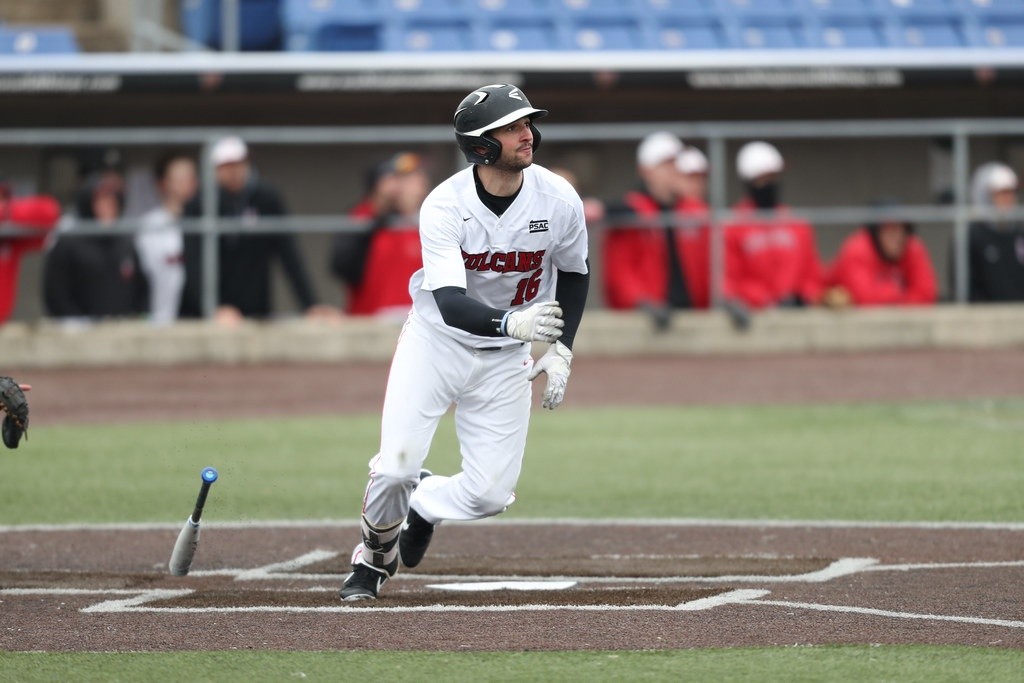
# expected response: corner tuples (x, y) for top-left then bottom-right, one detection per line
(337, 537), (398, 601)
(398, 469), (436, 570)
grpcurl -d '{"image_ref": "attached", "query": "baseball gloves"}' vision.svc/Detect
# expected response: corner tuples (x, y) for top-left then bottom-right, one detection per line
(0, 376), (30, 449)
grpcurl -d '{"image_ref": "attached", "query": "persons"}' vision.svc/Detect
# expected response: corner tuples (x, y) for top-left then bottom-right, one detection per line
(0, 375), (32, 449)
(0, 135), (430, 325)
(548, 130), (1024, 330)
(339, 84), (592, 601)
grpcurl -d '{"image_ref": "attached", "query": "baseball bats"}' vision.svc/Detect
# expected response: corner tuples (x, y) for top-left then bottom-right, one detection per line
(168, 467), (218, 575)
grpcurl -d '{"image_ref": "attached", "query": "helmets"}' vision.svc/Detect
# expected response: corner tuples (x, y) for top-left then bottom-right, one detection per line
(454, 83), (548, 165)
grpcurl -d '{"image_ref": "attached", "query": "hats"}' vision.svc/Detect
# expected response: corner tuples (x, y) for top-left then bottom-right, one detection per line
(639, 130), (680, 170)
(736, 142), (784, 178)
(677, 146), (709, 173)
(212, 137), (247, 165)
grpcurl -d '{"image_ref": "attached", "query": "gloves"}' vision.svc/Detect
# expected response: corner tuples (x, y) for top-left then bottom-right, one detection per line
(529, 338), (572, 410)
(504, 299), (564, 345)
